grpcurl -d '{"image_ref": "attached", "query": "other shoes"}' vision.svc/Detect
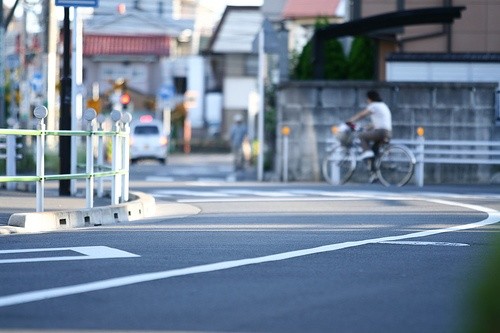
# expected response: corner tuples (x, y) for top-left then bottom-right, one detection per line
(233, 169), (245, 178)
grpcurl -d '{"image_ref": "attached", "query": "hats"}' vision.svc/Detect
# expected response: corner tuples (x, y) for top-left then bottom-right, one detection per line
(232, 113), (243, 122)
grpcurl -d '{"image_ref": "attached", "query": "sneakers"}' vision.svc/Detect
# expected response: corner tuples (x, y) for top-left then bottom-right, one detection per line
(356, 150), (375, 161)
(368, 172), (378, 184)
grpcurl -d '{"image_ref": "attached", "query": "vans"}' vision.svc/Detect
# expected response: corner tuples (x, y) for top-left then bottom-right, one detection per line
(81, 114), (167, 166)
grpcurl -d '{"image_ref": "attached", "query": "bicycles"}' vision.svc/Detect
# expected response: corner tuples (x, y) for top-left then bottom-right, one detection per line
(320, 122), (416, 187)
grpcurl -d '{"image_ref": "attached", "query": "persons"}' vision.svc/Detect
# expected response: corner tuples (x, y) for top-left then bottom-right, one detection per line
(346, 91), (392, 183)
